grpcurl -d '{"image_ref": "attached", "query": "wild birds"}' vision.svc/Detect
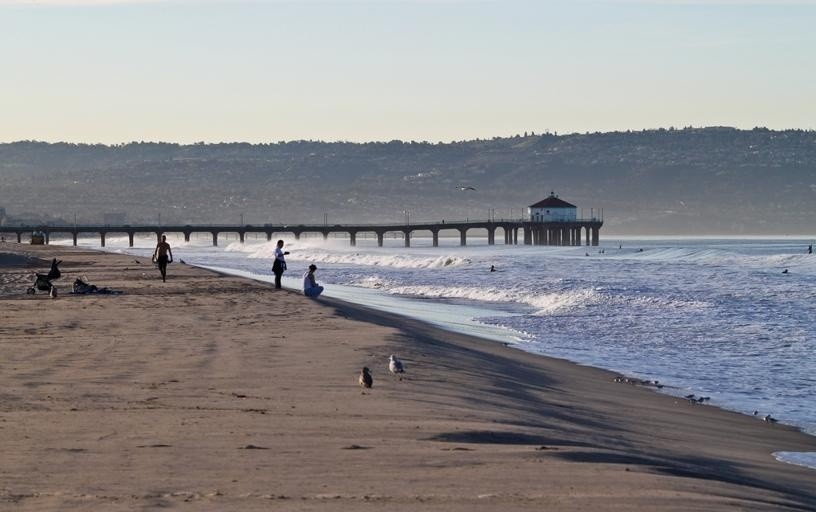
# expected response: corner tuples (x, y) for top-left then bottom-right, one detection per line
(684, 393), (711, 408)
(359, 366), (373, 388)
(456, 184), (477, 192)
(179, 258), (186, 264)
(388, 354), (406, 381)
(752, 409), (779, 424)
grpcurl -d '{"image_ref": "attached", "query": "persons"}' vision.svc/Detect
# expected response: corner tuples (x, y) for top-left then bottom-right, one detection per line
(154, 235), (173, 283)
(70, 278), (124, 295)
(271, 239), (291, 292)
(303, 264), (323, 299)
(32, 228), (43, 238)
